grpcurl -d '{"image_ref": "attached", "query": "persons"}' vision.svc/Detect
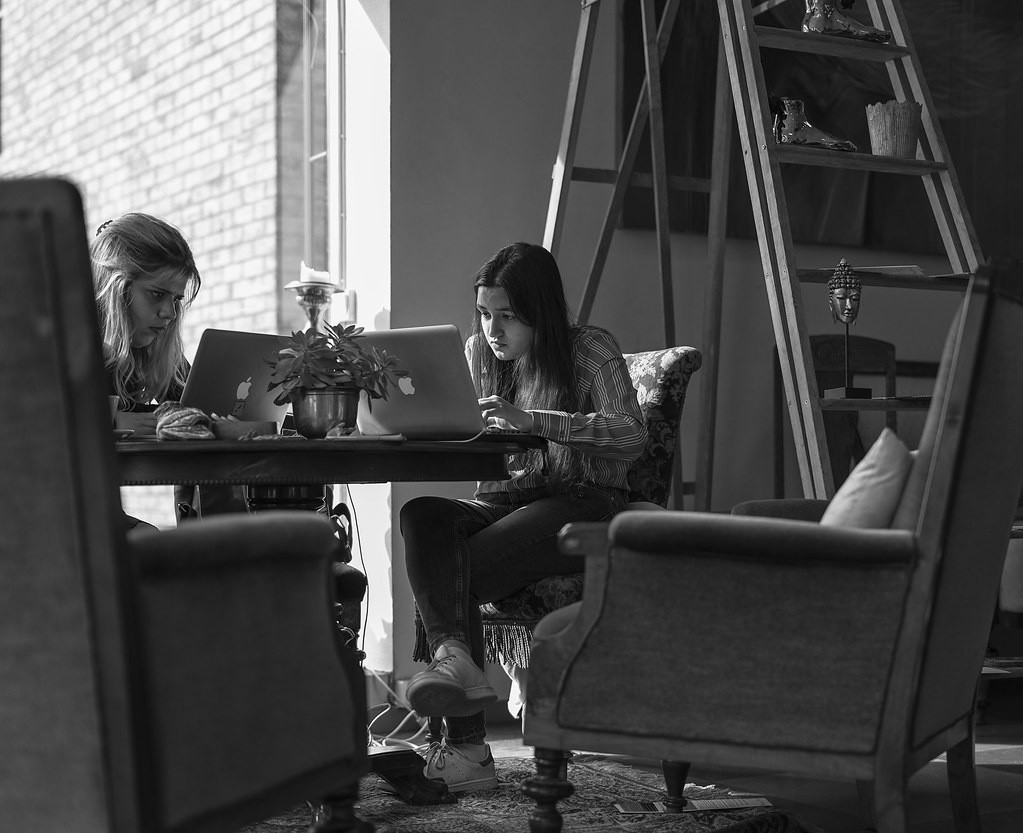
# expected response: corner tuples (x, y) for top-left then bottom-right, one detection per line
(801, 0), (892, 42)
(773, 96), (858, 151)
(376, 242), (649, 792)
(91, 211), (202, 528)
(827, 258), (863, 326)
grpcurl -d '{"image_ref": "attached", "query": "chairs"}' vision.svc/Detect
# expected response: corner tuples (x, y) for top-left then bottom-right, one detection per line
(520, 264), (1023, 833)
(413, 345), (703, 782)
(1, 176), (376, 833)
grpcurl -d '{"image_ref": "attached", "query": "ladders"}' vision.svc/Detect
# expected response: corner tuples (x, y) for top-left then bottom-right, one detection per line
(543, 0), (986, 500)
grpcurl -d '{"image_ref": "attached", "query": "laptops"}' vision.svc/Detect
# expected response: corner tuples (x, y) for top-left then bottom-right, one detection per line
(342, 323), (539, 441)
(178, 329), (296, 430)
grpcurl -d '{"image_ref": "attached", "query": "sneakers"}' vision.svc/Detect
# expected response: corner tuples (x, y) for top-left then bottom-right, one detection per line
(408, 645), (497, 717)
(420, 741), (499, 793)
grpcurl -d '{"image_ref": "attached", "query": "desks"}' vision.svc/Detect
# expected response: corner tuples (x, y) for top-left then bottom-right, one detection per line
(820, 398), (932, 411)
(115, 439), (526, 804)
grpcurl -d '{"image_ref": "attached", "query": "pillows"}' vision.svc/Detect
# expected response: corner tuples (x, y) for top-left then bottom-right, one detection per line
(821, 427), (919, 530)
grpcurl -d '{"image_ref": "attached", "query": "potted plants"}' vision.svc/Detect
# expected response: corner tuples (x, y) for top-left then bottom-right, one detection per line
(264, 319), (410, 439)
